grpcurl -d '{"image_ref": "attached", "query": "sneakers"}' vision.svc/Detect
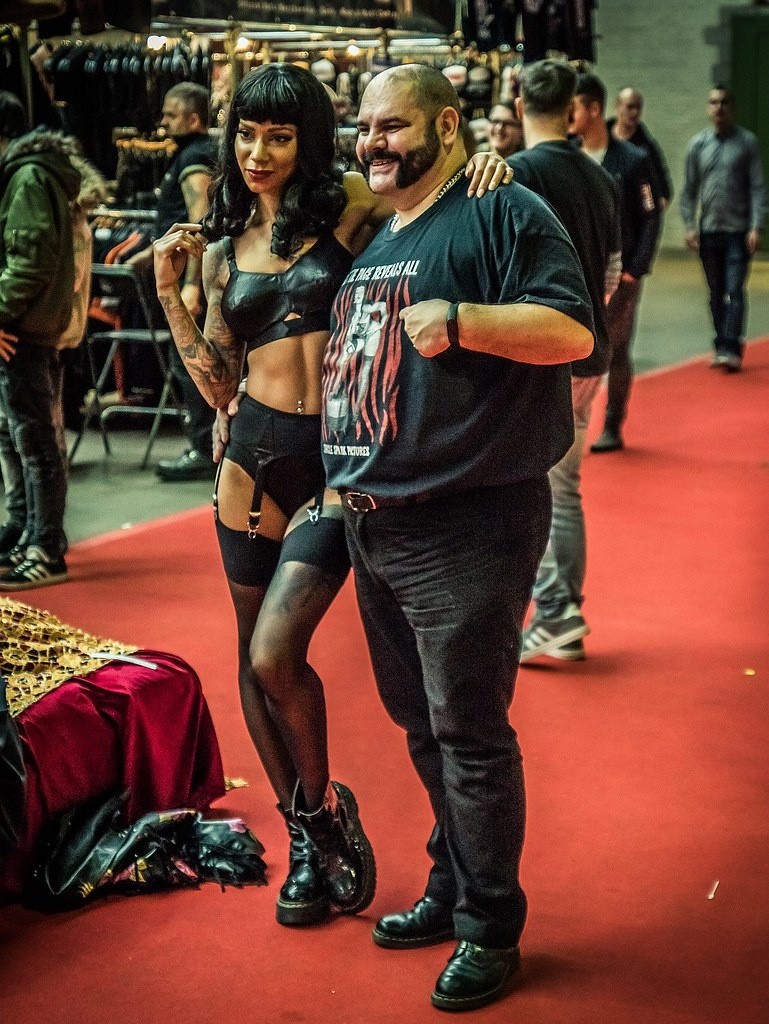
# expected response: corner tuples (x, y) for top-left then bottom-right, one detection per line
(545, 643), (590, 662)
(0, 543), (24, 576)
(520, 601), (590, 661)
(157, 446), (214, 482)
(0, 545), (68, 589)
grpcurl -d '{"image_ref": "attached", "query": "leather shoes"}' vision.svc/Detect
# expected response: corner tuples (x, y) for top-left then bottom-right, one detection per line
(370, 887), (454, 949)
(430, 938), (521, 1012)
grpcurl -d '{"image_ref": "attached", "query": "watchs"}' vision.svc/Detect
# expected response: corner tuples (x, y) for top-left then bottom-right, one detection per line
(445, 300), (464, 349)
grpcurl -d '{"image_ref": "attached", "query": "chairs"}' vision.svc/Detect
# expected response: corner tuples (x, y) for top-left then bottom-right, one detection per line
(67, 263), (187, 469)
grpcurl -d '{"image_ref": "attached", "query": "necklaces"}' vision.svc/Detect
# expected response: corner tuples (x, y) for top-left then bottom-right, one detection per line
(389, 165), (467, 236)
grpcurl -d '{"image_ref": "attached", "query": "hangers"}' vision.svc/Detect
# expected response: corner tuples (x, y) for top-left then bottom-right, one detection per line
(78, 209), (160, 248)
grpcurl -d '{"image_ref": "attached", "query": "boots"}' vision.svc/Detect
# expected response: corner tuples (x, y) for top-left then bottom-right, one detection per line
(288, 777), (377, 914)
(274, 802), (327, 926)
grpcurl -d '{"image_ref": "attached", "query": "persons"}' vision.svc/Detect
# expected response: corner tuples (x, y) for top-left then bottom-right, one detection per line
(215, 65), (610, 1017)
(154, 82), (239, 481)
(480, 97), (524, 159)
(149, 59), (516, 928)
(0, 96), (100, 588)
(604, 85), (675, 220)
(681, 85), (769, 375)
(564, 72), (665, 452)
(500, 56), (624, 664)
